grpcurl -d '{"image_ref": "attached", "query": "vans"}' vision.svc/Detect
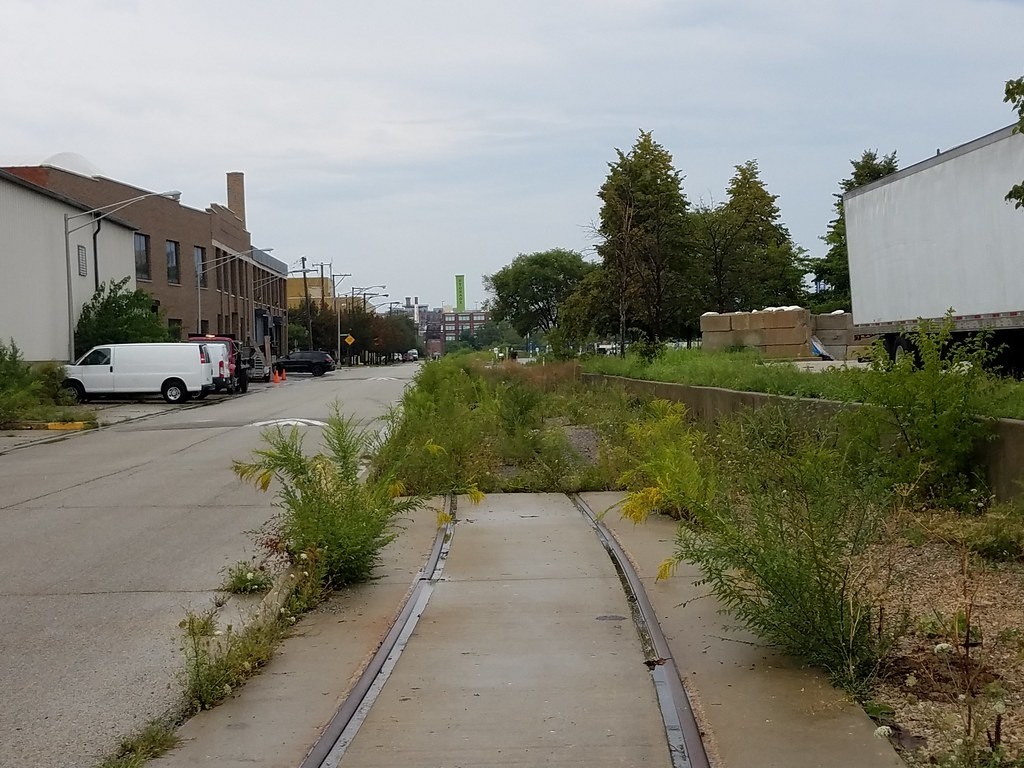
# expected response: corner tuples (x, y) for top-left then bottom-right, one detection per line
(206, 344), (230, 394)
(57, 343), (213, 406)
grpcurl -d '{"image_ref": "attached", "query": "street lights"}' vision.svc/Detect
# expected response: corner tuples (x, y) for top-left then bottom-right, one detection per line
(63, 190), (182, 364)
(251, 268), (310, 342)
(197, 247), (274, 334)
(350, 284), (390, 314)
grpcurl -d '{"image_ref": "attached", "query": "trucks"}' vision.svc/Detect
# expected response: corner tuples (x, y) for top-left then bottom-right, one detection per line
(408, 349), (418, 361)
(187, 334), (248, 395)
(842, 121), (1024, 380)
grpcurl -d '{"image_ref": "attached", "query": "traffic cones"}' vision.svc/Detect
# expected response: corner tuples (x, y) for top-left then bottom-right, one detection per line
(281, 368), (286, 381)
(274, 370), (281, 383)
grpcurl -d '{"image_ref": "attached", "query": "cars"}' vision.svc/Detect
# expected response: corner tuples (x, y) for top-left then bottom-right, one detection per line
(402, 353), (414, 363)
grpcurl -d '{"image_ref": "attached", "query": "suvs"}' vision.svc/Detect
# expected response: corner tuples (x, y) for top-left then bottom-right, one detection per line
(272, 351), (336, 377)
(381, 353), (403, 362)
